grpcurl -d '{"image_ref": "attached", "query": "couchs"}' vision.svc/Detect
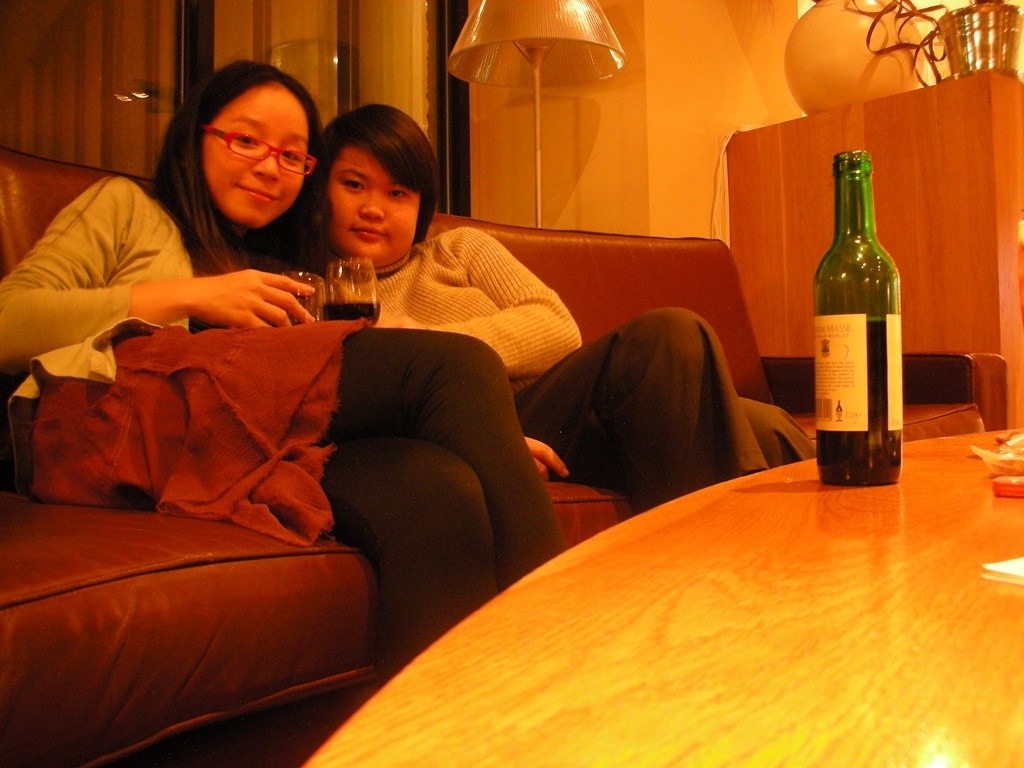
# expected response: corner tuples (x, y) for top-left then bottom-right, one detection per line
(0, 148), (985, 768)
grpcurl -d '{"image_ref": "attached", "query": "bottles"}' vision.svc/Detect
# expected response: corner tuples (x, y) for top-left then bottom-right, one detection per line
(813, 150), (902, 484)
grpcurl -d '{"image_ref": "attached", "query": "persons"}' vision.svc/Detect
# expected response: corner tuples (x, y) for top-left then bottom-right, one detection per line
(0, 58), (568, 687)
(250, 104), (817, 511)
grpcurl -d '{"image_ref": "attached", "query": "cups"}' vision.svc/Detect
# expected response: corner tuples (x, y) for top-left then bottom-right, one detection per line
(278, 271), (325, 325)
(325, 257), (380, 327)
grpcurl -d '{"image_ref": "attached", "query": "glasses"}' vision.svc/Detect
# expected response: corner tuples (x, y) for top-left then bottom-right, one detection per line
(202, 124), (317, 176)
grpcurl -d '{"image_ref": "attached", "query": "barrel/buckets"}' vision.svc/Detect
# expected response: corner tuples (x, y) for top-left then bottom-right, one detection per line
(939, 4), (1024, 82)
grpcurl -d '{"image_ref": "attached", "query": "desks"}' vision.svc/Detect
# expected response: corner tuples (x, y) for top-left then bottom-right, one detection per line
(299, 428), (1024, 768)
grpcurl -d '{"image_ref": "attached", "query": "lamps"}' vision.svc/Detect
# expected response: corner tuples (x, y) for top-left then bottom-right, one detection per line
(446, 0), (629, 229)
